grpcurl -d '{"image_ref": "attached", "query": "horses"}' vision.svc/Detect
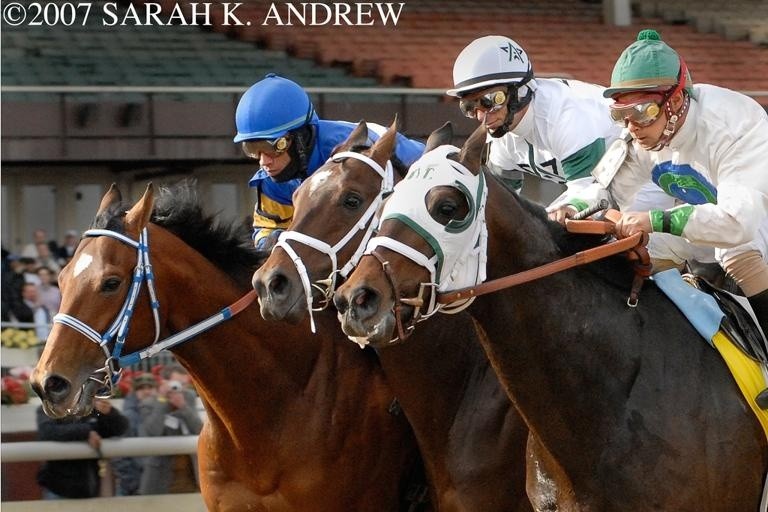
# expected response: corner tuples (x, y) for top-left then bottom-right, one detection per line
(23, 108), (768, 512)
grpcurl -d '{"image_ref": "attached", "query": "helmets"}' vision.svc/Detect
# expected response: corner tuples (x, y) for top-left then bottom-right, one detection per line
(446, 35), (538, 97)
(233, 73), (320, 144)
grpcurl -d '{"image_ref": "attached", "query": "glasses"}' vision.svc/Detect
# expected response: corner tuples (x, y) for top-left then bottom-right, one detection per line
(608, 98), (673, 128)
(459, 85), (510, 118)
(242, 132), (291, 159)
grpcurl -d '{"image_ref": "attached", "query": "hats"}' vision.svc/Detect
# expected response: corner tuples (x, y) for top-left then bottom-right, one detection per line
(134, 374), (159, 387)
(603, 28), (695, 98)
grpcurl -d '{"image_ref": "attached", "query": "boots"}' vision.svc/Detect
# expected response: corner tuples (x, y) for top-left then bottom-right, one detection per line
(747, 288), (768, 409)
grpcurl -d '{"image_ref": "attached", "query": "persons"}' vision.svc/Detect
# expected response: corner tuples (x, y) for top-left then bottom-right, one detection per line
(2, 228), (204, 497)
(546, 30), (768, 344)
(233, 72), (426, 253)
(445, 34), (624, 195)
